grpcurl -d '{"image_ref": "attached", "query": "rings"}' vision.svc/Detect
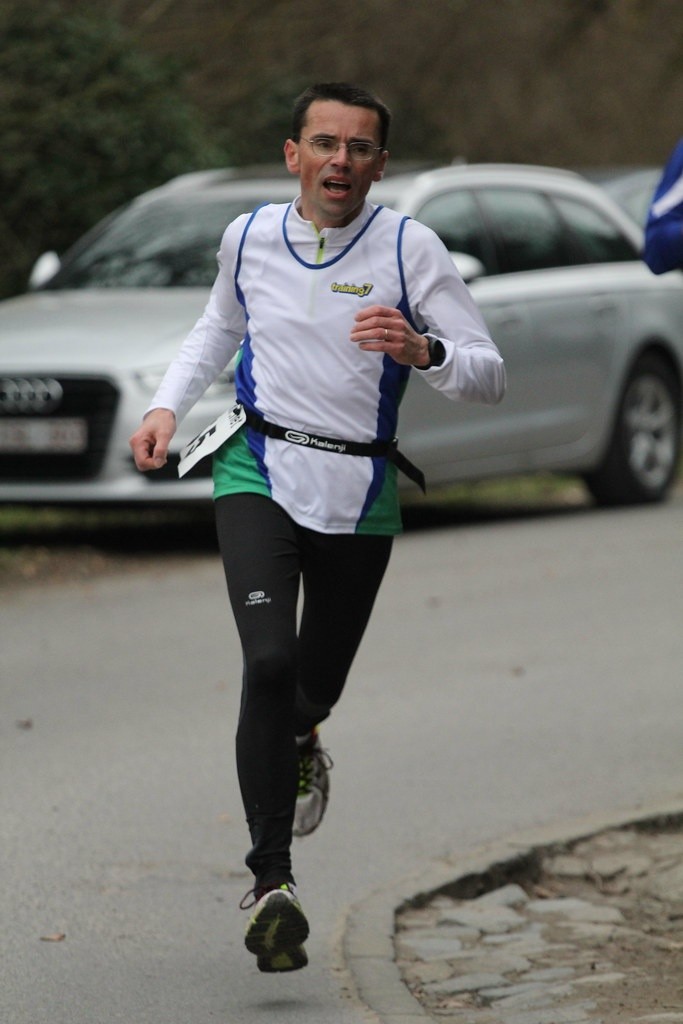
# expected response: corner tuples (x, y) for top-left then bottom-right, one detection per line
(384, 328), (389, 341)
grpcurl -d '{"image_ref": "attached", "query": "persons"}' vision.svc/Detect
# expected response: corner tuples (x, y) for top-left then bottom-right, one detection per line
(131, 84), (508, 973)
(641, 138), (683, 275)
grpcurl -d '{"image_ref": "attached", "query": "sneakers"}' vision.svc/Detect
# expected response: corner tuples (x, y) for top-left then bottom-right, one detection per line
(293, 728), (334, 836)
(239, 880), (310, 974)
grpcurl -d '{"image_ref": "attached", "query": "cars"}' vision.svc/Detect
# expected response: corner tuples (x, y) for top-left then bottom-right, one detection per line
(0, 157), (683, 527)
(595, 168), (666, 242)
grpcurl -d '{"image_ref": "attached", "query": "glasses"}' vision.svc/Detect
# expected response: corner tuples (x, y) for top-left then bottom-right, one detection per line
(298, 135), (383, 162)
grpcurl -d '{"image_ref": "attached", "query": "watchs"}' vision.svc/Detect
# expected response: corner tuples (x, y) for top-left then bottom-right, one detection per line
(412, 333), (446, 371)
